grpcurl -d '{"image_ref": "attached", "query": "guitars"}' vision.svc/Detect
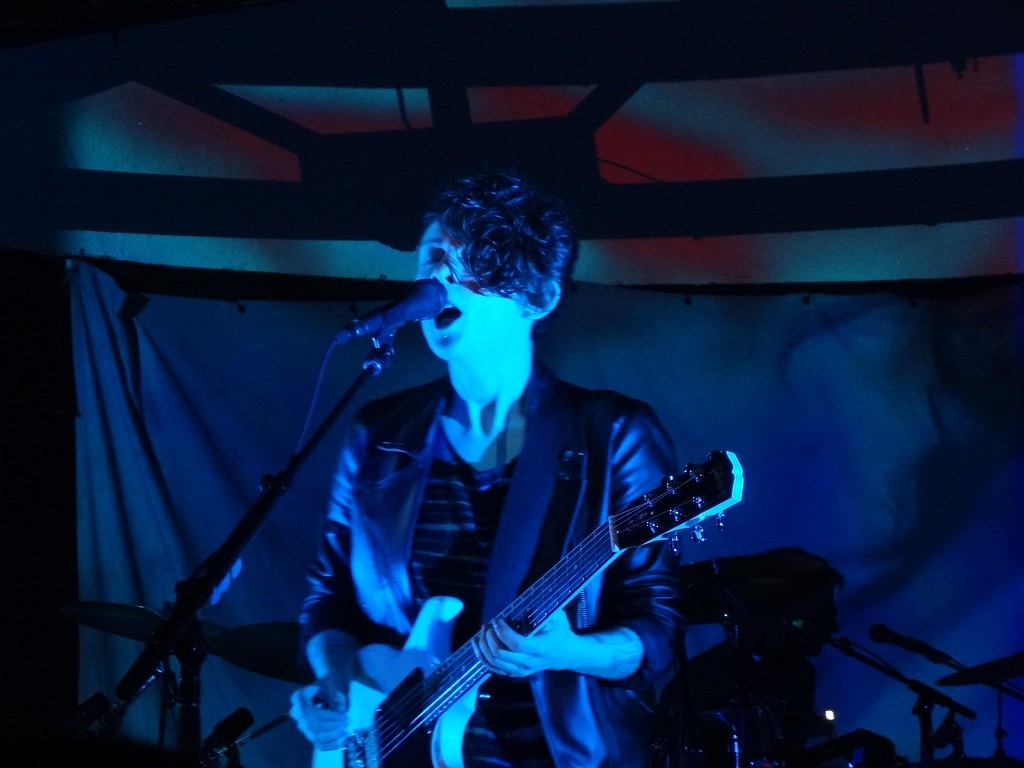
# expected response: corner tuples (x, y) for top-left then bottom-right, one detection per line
(310, 452), (747, 768)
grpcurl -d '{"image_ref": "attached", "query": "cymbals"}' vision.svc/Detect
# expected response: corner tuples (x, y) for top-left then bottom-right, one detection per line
(936, 651), (1022, 687)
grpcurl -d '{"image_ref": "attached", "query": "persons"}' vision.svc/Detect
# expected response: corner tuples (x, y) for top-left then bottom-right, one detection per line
(288, 179), (685, 768)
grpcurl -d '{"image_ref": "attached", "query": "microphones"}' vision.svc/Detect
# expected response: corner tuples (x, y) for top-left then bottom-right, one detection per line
(334, 278), (447, 341)
